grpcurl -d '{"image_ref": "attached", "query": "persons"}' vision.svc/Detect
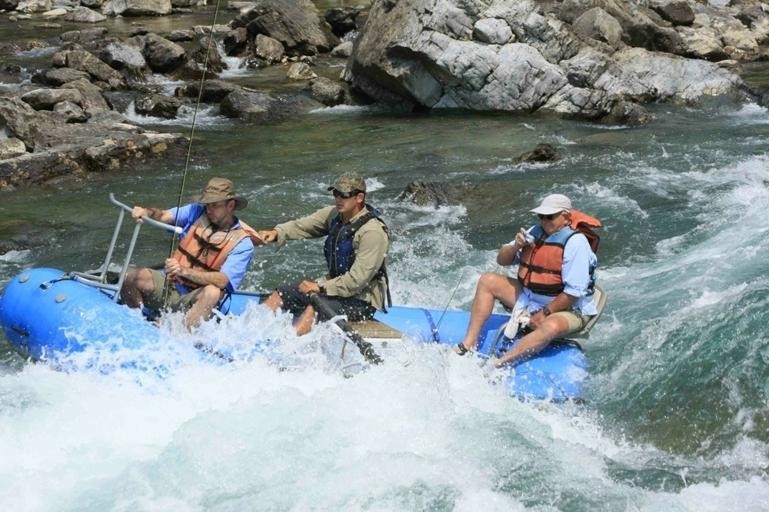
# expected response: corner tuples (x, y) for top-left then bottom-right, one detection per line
(117, 175), (262, 331)
(454, 193), (600, 372)
(254, 171), (393, 336)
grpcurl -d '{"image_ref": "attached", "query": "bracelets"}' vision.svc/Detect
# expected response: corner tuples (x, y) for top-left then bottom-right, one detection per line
(316, 280), (325, 293)
(542, 305), (551, 317)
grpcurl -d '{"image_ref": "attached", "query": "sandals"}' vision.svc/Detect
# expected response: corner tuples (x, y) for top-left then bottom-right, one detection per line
(453, 342), (466, 356)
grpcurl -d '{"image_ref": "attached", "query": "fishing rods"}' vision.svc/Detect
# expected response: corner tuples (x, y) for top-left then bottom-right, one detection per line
(159, 1), (221, 314)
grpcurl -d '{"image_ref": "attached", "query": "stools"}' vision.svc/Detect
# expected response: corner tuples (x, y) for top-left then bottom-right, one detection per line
(314, 316), (403, 377)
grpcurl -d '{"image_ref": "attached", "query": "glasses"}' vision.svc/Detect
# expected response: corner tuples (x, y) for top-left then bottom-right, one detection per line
(537, 214), (553, 220)
(332, 189), (360, 198)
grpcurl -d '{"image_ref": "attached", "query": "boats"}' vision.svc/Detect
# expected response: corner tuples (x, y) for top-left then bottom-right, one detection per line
(0, 267), (589, 403)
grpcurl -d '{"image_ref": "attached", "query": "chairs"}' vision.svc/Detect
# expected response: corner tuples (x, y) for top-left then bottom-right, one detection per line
(486, 284), (608, 362)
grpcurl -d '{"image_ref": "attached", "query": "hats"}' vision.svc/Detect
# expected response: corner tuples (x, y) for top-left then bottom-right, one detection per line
(328, 172), (366, 193)
(528, 194), (572, 215)
(198, 176), (248, 210)
